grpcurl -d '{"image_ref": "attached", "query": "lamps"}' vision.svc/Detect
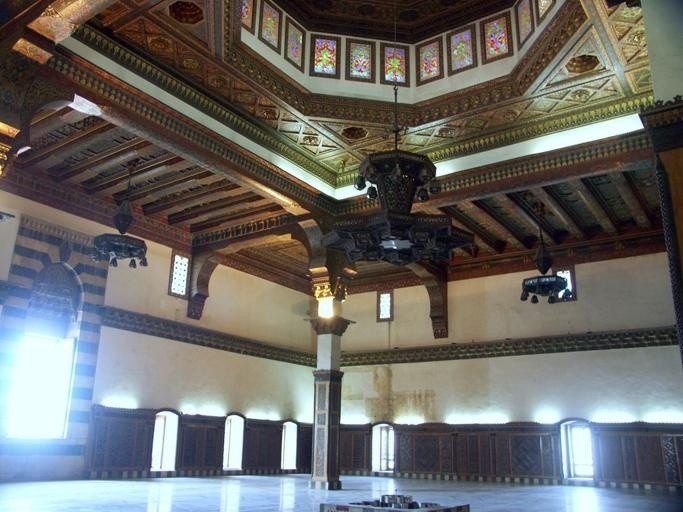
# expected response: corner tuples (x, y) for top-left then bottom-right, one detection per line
(320, 5), (474, 267)
(520, 202), (572, 303)
(91, 161), (148, 268)
